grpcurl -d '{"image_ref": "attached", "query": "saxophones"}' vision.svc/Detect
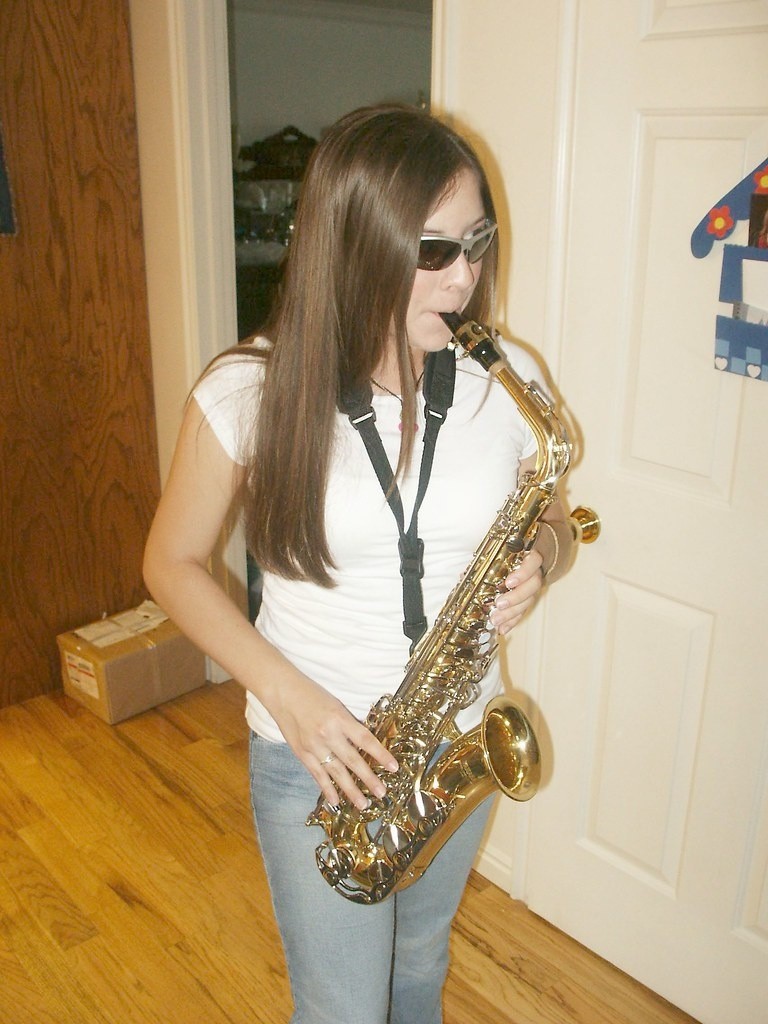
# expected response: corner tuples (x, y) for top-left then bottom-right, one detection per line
(301, 311), (603, 907)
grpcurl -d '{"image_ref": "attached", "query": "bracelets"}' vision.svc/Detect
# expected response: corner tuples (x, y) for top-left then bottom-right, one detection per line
(541, 521), (559, 579)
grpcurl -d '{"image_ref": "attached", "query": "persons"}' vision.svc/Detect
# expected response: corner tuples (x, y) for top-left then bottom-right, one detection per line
(142, 101), (572, 1024)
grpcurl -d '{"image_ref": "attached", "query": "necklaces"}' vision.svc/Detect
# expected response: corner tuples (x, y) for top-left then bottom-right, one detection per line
(368, 371), (424, 434)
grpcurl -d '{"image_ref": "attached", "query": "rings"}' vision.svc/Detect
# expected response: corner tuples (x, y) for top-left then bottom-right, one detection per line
(320, 752), (338, 765)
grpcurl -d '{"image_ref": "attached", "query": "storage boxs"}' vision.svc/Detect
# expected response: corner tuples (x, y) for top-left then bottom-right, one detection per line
(58, 602), (206, 725)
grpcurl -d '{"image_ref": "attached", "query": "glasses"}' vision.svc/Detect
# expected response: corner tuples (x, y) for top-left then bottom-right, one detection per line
(417, 219), (498, 271)
